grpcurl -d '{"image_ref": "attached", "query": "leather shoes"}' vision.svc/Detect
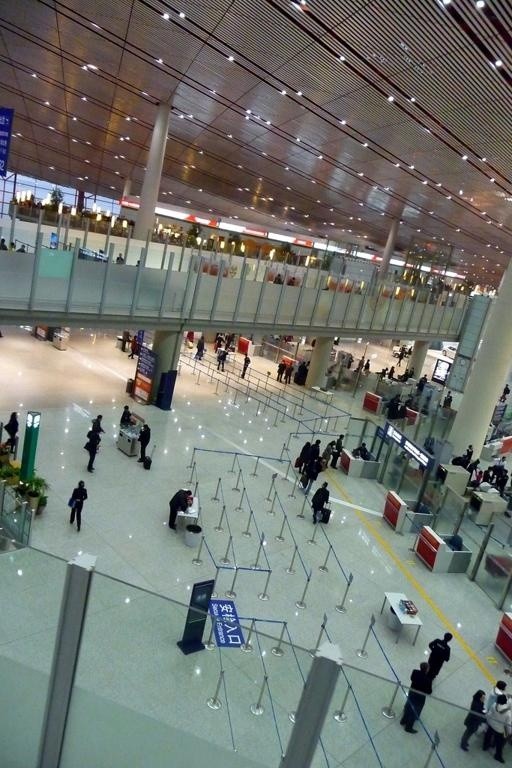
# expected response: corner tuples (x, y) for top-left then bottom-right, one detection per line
(405, 726), (418, 734)
(400, 718), (406, 725)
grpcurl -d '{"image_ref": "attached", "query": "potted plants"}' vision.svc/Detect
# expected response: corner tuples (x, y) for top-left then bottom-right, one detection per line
(0, 462), (49, 515)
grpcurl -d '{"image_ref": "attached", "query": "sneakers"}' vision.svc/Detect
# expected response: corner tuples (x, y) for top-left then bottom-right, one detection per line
(137, 458), (144, 462)
(494, 753), (504, 763)
(461, 743), (470, 751)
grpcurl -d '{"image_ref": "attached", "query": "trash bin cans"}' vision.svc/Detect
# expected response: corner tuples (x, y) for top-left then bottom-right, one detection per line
(52, 332), (69, 350)
(126, 378), (134, 393)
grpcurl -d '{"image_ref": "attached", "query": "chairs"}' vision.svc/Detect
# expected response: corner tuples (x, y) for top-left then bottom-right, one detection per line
(442, 534), (462, 551)
(412, 503), (430, 513)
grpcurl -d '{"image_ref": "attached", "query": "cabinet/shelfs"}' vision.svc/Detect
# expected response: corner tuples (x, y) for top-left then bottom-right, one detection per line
(118, 428), (139, 457)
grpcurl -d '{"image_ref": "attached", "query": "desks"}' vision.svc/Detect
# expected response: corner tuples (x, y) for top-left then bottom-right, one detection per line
(175, 496), (198, 532)
(379, 592), (423, 645)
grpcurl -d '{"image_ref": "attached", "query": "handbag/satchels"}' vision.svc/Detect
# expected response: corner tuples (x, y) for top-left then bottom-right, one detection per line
(452, 457), (465, 466)
(504, 726), (508, 738)
(295, 457), (304, 468)
(84, 441), (91, 451)
(300, 474), (309, 489)
(68, 498), (76, 508)
(4, 423), (9, 431)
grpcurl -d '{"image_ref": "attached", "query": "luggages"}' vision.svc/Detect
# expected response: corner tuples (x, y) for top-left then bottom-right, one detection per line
(143, 444), (157, 470)
(320, 501), (332, 524)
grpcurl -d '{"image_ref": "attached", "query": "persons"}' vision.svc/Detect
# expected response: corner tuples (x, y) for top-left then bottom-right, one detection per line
(347, 344), (452, 420)
(294, 433), (369, 496)
(128, 336), (138, 359)
(116, 252), (123, 263)
(84, 428), (102, 473)
(400, 662), (433, 733)
(312, 481), (330, 524)
(4, 412), (19, 453)
(0, 238), (26, 253)
(137, 424), (150, 462)
(459, 681), (512, 764)
(120, 404), (134, 423)
(286, 277), (295, 286)
(276, 359), (310, 385)
(428, 631), (453, 680)
(499, 384), (510, 402)
(68, 479), (88, 532)
(92, 413), (106, 444)
(273, 273), (283, 284)
(452, 444), (512, 499)
(168, 488), (192, 530)
(185, 330), (254, 380)
